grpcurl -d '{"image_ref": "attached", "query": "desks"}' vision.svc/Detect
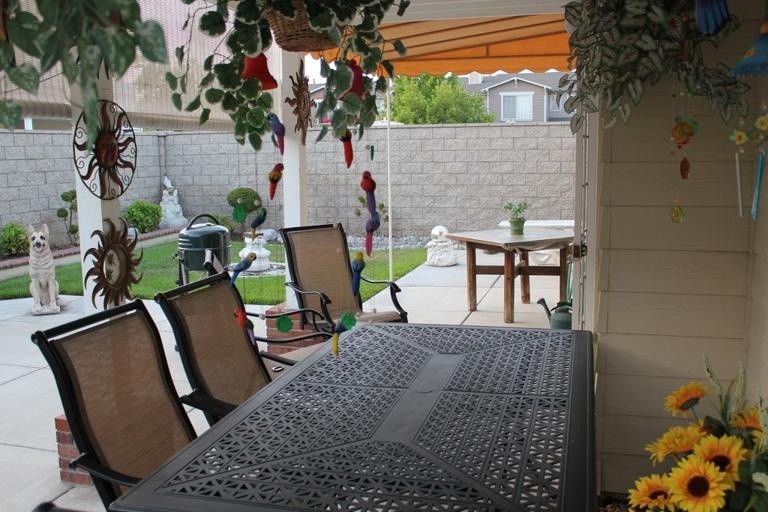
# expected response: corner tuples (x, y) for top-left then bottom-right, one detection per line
(445, 228), (576, 324)
(103, 320), (595, 508)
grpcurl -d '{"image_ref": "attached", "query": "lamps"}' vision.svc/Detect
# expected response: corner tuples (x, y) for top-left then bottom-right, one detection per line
(336, 59), (365, 100)
(240, 51), (277, 91)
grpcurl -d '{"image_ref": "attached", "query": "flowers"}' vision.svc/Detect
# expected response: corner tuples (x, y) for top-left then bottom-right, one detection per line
(627, 354), (768, 512)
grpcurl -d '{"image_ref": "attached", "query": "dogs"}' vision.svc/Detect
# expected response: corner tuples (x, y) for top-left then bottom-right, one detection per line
(26, 222), (60, 312)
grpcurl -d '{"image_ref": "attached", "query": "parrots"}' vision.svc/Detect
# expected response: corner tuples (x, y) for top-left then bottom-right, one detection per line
(228, 113), (287, 346)
(328, 127), (381, 359)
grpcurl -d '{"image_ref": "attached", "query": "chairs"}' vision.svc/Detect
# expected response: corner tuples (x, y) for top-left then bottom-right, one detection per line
(155, 271), (298, 427)
(276, 223), (408, 334)
(203, 248), (336, 361)
(32, 298), (237, 510)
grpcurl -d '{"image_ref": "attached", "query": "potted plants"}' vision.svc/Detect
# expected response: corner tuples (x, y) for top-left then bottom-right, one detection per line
(502, 199), (527, 236)
